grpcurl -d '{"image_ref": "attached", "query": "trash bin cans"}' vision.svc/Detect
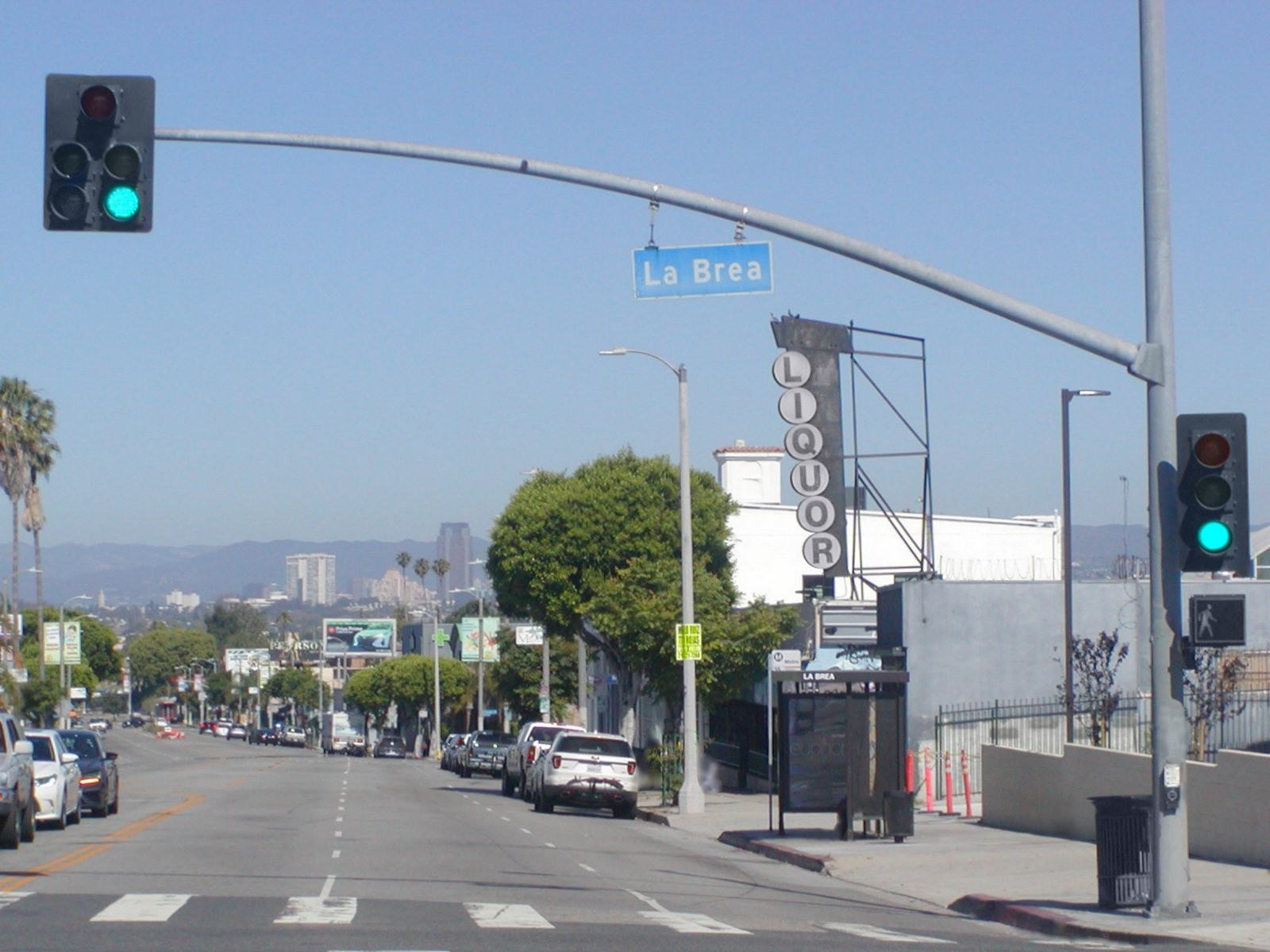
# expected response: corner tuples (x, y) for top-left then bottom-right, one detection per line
(1085, 794), (1156, 912)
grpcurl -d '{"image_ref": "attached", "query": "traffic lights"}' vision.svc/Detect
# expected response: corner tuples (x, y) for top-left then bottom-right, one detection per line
(42, 73), (155, 234)
(1189, 594), (1249, 646)
(1168, 412), (1253, 575)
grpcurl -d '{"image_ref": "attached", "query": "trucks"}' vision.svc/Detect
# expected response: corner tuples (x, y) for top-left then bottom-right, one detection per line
(318, 712), (367, 757)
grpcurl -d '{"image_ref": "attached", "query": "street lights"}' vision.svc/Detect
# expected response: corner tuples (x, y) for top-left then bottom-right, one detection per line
(408, 610), (441, 761)
(450, 588), (484, 730)
(595, 346), (706, 817)
(67, 613), (100, 704)
(1059, 389), (1116, 742)
(60, 596), (92, 720)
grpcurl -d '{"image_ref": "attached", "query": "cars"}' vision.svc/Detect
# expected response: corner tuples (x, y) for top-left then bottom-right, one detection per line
(24, 728), (83, 831)
(531, 731), (640, 821)
(0, 713), (35, 850)
(438, 729), (518, 779)
(65, 712), (187, 733)
(373, 735), (406, 759)
(198, 714), (307, 748)
(502, 721), (587, 800)
(53, 728), (119, 819)
